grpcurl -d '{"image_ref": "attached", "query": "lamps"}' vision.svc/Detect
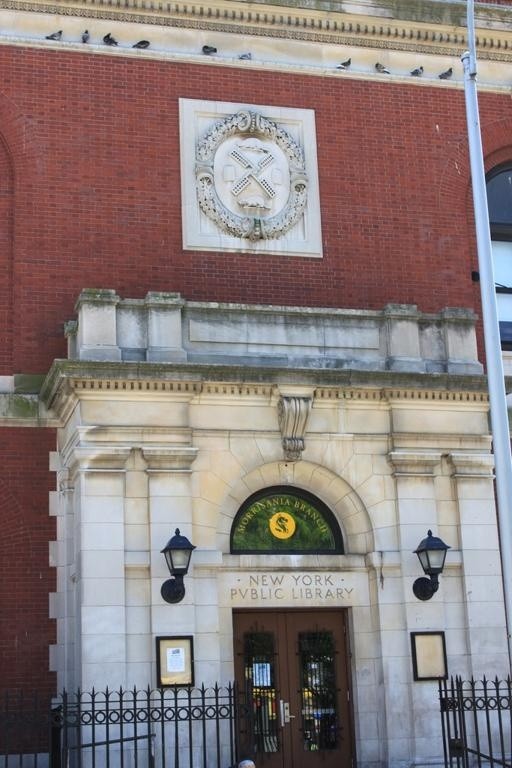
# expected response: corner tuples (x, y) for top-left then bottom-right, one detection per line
(161, 527), (197, 603)
(413, 530), (452, 601)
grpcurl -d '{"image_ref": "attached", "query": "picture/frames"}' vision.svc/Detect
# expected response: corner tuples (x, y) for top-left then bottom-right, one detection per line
(156, 636), (194, 687)
(411, 632), (448, 681)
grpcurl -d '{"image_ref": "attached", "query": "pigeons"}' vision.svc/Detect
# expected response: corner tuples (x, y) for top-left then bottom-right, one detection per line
(103, 33), (118, 46)
(202, 45), (217, 55)
(375, 62), (391, 74)
(79, 30), (90, 43)
(238, 52), (252, 60)
(410, 66), (424, 76)
(336, 58), (351, 69)
(46, 30), (63, 40)
(438, 67), (452, 79)
(132, 40), (150, 48)
